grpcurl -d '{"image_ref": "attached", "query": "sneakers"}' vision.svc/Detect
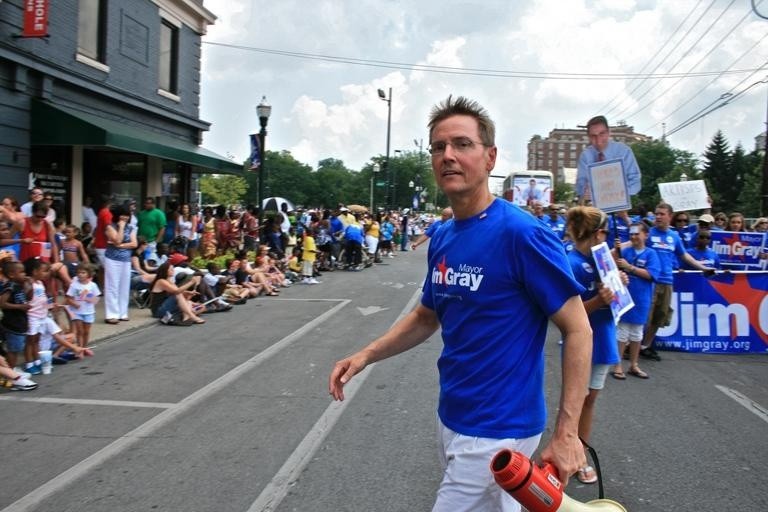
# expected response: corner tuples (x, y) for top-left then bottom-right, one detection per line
(33, 359), (42, 370)
(58, 351), (80, 361)
(50, 356), (68, 365)
(285, 279), (292, 284)
(78, 351), (85, 360)
(374, 259), (383, 263)
(623, 343), (631, 361)
(214, 298), (232, 313)
(120, 317), (130, 322)
(10, 379), (40, 391)
(105, 318), (121, 325)
(390, 253), (398, 256)
(182, 317), (192, 323)
(12, 367), (33, 379)
(233, 298), (247, 305)
(84, 349), (95, 357)
(281, 283), (292, 288)
(640, 346), (662, 362)
(308, 277), (320, 284)
(400, 248), (408, 251)
(301, 278), (310, 283)
(313, 261), (360, 276)
(24, 363), (42, 375)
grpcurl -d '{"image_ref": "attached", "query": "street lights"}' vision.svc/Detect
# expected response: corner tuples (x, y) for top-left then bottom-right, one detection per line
(378, 87), (392, 218)
(373, 161), (380, 217)
(408, 179), (414, 208)
(257, 96), (273, 232)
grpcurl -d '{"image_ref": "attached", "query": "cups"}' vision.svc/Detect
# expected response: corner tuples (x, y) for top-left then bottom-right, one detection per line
(39, 351), (54, 375)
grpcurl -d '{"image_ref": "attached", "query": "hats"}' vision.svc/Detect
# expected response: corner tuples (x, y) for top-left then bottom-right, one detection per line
(169, 252), (190, 265)
(258, 244), (272, 251)
(174, 271), (194, 288)
(697, 214), (715, 223)
(403, 208), (410, 215)
(339, 207), (348, 212)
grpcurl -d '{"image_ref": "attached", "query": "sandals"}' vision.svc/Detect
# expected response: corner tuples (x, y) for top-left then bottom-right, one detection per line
(613, 366), (624, 380)
(577, 462), (600, 486)
(629, 365), (649, 378)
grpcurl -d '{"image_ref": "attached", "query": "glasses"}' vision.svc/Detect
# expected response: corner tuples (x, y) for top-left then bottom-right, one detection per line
(428, 135), (492, 154)
(698, 234), (711, 240)
(759, 222), (768, 225)
(35, 213), (45, 219)
(601, 229), (610, 236)
(716, 217), (726, 221)
(549, 208), (560, 211)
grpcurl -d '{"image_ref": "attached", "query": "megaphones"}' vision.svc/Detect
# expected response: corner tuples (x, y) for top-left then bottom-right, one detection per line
(490, 438), (627, 512)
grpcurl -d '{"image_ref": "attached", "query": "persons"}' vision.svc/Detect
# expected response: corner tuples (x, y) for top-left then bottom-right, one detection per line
(130, 196), (435, 327)
(521, 180), (543, 203)
(327, 91), (595, 512)
(1, 187), (139, 392)
(411, 208), (453, 251)
(574, 116), (642, 206)
(513, 185), (767, 379)
(560, 204), (629, 485)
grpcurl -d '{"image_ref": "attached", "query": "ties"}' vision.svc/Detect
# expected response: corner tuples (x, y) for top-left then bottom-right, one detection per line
(597, 152), (606, 162)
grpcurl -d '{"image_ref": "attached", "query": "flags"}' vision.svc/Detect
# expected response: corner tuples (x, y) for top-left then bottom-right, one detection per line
(248, 133), (262, 172)
(21, 0), (49, 38)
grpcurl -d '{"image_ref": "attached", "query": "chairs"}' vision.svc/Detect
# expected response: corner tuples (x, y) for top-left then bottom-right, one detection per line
(130, 286), (151, 309)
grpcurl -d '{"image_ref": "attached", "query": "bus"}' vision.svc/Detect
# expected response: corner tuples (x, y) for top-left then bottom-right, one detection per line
(502, 169), (554, 208)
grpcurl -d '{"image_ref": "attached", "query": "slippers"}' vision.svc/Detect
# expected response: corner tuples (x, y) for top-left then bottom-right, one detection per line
(272, 287), (281, 292)
(265, 291), (279, 296)
(192, 317), (205, 323)
